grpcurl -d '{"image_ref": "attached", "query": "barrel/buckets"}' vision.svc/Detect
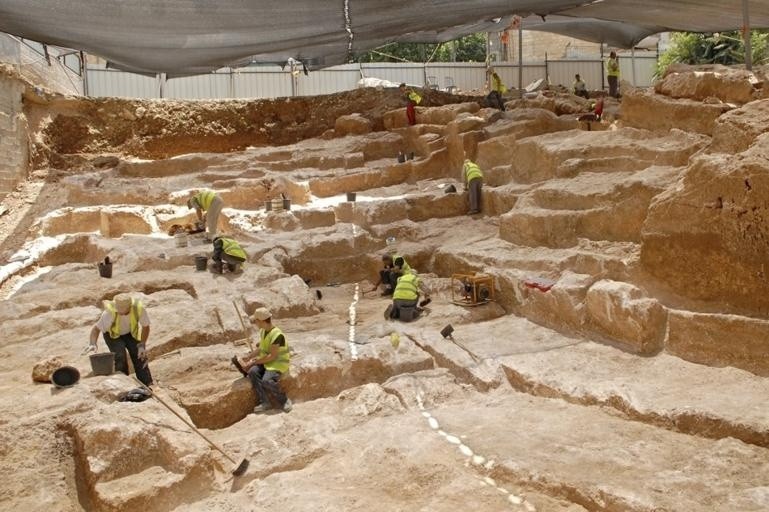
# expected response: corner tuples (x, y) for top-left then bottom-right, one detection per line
(98, 263), (112, 277)
(175, 234), (188, 247)
(283, 200), (291, 209)
(196, 256), (207, 270)
(90, 353), (115, 375)
(264, 201), (272, 210)
(398, 154), (405, 162)
(272, 197), (283, 211)
(51, 367), (80, 388)
(444, 186), (456, 193)
(387, 237), (398, 253)
(347, 192), (355, 201)
(407, 152), (414, 160)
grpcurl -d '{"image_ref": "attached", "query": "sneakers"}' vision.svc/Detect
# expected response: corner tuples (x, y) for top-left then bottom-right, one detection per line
(383, 303), (393, 318)
(253, 402), (272, 413)
(282, 397), (292, 412)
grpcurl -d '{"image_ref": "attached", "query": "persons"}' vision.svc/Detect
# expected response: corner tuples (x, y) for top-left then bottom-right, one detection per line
(187, 190), (224, 242)
(461, 157), (484, 214)
(211, 233), (247, 274)
(572, 73), (590, 100)
(486, 64), (505, 110)
(372, 251), (412, 295)
(703, 32), (730, 64)
(383, 268), (432, 320)
(241, 307), (291, 413)
(607, 50), (620, 98)
(81, 293), (153, 386)
(399, 82), (422, 126)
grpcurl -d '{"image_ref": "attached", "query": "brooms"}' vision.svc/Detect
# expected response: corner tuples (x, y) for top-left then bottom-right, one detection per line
(133, 376), (249, 476)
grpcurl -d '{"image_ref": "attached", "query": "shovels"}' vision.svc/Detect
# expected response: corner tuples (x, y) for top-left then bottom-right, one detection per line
(440, 324), (479, 359)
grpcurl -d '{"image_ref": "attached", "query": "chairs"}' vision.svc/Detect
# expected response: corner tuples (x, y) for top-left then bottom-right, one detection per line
(444, 76), (458, 95)
(426, 75), (439, 91)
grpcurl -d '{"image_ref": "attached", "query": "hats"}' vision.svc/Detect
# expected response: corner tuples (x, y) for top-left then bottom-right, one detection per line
(250, 307), (272, 321)
(113, 293), (132, 314)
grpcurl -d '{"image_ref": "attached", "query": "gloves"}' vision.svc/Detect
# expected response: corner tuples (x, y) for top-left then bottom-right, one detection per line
(81, 345), (98, 356)
(135, 343), (146, 362)
